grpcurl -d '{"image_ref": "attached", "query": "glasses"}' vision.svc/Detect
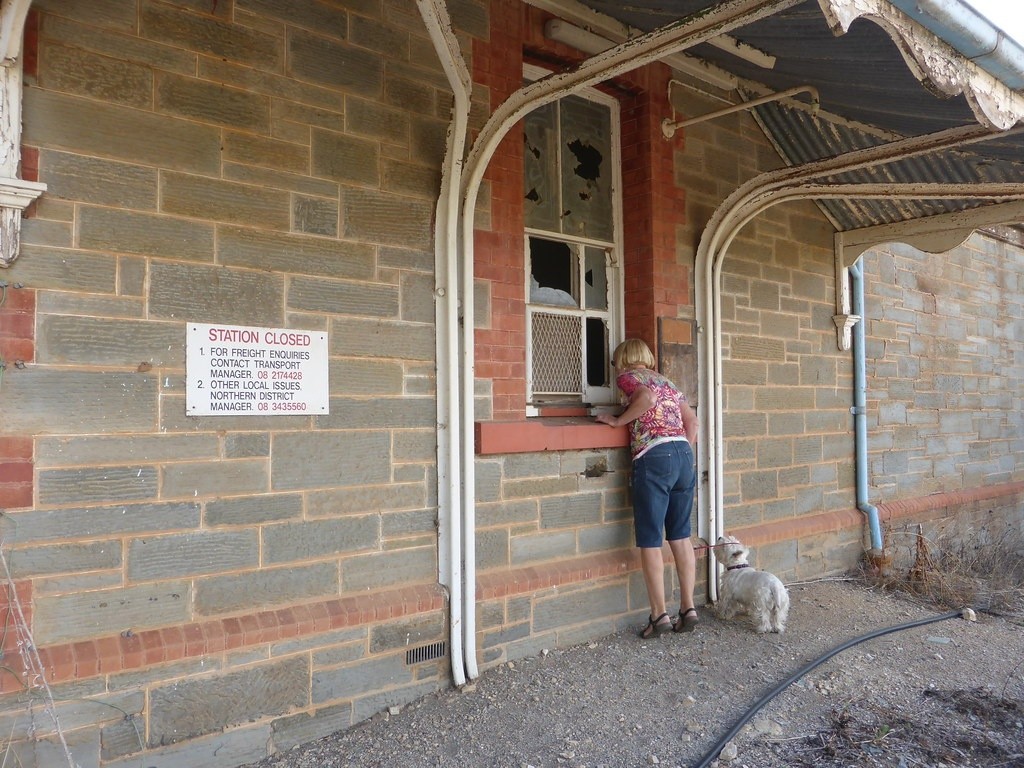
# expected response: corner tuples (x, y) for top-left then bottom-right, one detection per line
(610, 360), (615, 367)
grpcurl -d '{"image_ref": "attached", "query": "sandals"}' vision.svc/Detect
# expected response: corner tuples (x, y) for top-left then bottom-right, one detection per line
(641, 613), (673, 639)
(672, 608), (699, 632)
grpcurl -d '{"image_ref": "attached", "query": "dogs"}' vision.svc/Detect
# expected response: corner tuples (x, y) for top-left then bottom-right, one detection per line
(712, 535), (790, 634)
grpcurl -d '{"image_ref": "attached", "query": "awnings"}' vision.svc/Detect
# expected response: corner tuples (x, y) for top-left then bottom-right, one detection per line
(415, 0), (1024, 254)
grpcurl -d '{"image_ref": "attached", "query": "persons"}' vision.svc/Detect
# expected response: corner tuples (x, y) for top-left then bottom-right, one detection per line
(593, 338), (700, 638)
